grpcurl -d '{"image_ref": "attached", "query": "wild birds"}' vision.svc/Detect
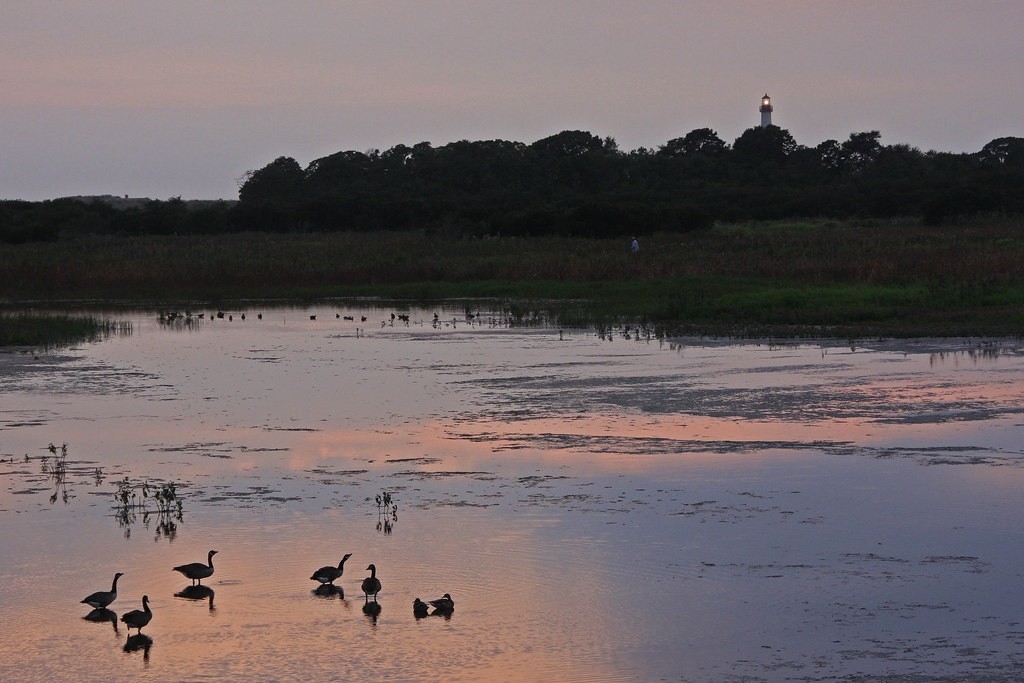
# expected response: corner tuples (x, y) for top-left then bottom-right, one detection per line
(172, 549), (220, 587)
(80, 572), (124, 613)
(412, 593), (456, 620)
(153, 305), (512, 329)
(310, 553), (355, 589)
(119, 594), (157, 636)
(360, 563), (385, 602)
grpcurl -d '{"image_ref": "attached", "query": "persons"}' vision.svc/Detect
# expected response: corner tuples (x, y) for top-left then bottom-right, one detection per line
(631, 237), (639, 253)
